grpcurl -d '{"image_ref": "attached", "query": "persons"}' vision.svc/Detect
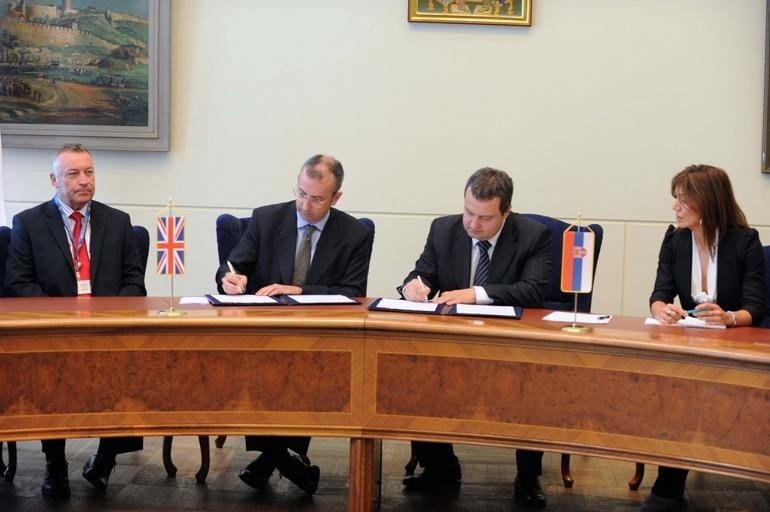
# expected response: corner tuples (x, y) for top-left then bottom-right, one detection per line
(642, 161), (770, 511)
(209, 152), (373, 498)
(396, 163), (550, 507)
(4, 143), (146, 504)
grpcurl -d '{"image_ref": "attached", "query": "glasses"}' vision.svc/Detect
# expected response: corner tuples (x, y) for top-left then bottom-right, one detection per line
(292, 188), (327, 205)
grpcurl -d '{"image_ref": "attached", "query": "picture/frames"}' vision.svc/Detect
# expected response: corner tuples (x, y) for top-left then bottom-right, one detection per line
(407, 0), (532, 29)
(0, 0), (174, 155)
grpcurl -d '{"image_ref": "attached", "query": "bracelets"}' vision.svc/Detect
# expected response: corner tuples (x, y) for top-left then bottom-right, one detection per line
(727, 311), (738, 327)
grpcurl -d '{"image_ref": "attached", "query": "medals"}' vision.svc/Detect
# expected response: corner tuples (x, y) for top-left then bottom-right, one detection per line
(74, 260), (82, 271)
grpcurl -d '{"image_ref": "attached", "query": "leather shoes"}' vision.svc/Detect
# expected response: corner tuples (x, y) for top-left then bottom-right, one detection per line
(279, 452), (321, 496)
(638, 478), (686, 511)
(513, 471), (547, 506)
(82, 447), (117, 491)
(402, 458), (462, 486)
(41, 457), (72, 504)
(238, 451), (276, 491)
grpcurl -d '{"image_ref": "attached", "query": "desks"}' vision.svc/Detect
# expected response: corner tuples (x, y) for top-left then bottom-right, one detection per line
(0, 288), (768, 512)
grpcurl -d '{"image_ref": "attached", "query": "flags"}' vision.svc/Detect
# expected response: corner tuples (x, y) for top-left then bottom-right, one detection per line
(560, 230), (594, 295)
(157, 215), (187, 277)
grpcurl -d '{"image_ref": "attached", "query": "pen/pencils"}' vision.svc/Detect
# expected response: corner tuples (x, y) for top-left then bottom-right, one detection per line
(683, 308), (703, 314)
(594, 316), (610, 319)
(417, 275), (428, 303)
(226, 259), (246, 293)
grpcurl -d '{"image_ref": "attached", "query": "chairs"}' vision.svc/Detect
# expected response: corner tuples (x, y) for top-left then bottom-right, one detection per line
(0, 222), (179, 486)
(625, 243), (768, 493)
(400, 212), (604, 491)
(193, 213), (375, 489)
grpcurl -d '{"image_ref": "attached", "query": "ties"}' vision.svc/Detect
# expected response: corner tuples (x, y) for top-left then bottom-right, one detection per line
(70, 211), (93, 296)
(291, 223), (316, 288)
(472, 239), (492, 286)
